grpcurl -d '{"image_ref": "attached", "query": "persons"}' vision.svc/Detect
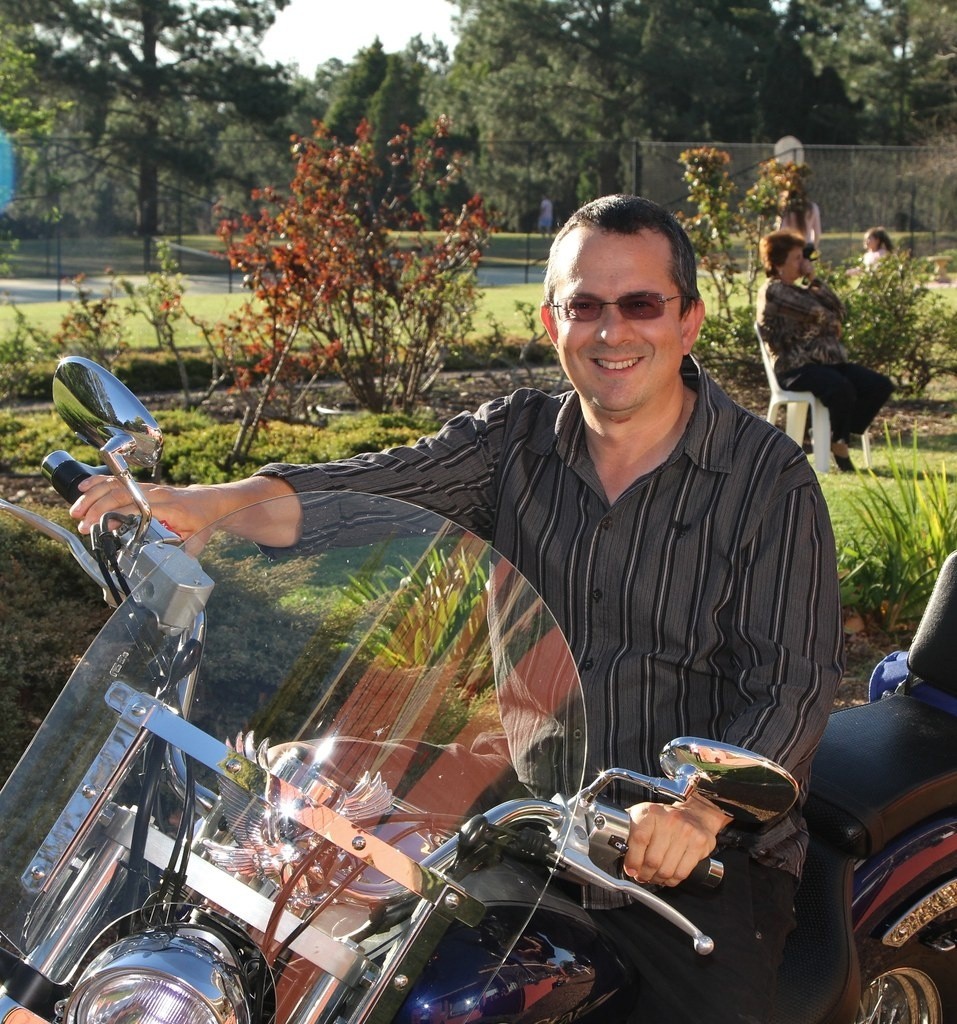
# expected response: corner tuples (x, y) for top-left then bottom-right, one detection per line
(539, 194), (552, 238)
(757, 229), (895, 474)
(68, 194), (846, 1024)
(845, 227), (894, 276)
(774, 179), (821, 263)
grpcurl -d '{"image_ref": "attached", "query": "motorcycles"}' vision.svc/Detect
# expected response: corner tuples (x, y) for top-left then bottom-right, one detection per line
(0, 355), (957, 1024)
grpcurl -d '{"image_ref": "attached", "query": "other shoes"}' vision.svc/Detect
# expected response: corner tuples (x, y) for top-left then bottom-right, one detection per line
(832, 447), (856, 475)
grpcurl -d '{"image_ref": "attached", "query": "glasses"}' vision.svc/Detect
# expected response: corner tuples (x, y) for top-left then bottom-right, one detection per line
(555, 289), (680, 321)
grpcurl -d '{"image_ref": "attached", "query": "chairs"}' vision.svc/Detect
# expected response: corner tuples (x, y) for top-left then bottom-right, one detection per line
(754, 321), (874, 473)
